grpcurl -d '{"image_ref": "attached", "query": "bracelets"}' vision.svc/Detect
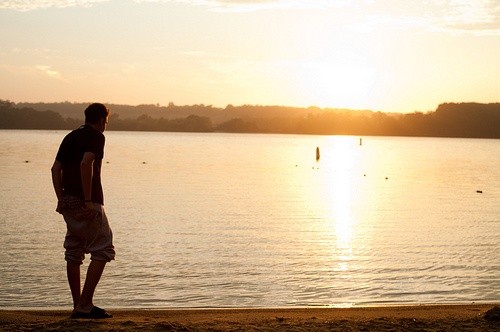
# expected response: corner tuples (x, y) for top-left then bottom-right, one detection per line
(82, 198), (92, 203)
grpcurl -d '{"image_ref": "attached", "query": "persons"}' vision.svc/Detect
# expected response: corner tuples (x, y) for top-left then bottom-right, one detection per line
(51, 103), (116, 319)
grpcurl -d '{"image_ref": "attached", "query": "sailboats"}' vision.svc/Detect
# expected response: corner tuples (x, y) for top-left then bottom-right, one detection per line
(360, 138), (363, 145)
(312, 146), (321, 170)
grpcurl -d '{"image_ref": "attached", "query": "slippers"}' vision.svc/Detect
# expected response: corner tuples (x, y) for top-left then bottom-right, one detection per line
(72, 306), (113, 319)
